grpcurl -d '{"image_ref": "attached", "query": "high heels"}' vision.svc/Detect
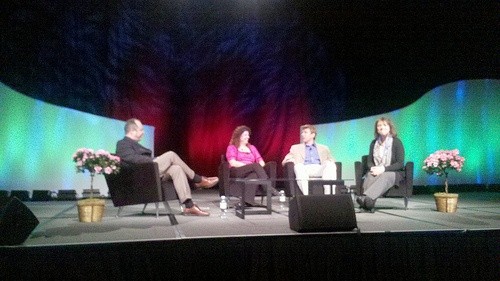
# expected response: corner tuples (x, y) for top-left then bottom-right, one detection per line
(356, 196), (375, 213)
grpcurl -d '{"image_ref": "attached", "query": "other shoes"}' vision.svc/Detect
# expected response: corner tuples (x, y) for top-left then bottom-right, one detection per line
(271, 187), (282, 196)
(245, 201), (260, 207)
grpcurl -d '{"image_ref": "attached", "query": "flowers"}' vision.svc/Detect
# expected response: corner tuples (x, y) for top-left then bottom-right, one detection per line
(421, 148), (465, 193)
(72, 147), (121, 198)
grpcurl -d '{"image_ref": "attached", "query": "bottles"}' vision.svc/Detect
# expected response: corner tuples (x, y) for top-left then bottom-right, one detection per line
(279, 190), (287, 212)
(220, 195), (228, 219)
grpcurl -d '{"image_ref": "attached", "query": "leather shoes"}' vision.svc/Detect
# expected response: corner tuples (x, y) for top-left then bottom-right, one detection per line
(195, 176), (219, 191)
(184, 204), (210, 216)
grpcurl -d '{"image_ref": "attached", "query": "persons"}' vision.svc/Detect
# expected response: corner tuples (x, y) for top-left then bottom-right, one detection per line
(226, 125), (280, 206)
(354, 116), (405, 212)
(281, 123), (337, 199)
(114, 116), (219, 218)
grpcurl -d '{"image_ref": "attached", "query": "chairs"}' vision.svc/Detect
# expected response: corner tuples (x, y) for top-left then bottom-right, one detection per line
(218, 155), (277, 212)
(355, 154), (414, 210)
(283, 161), (341, 207)
(102, 154), (184, 220)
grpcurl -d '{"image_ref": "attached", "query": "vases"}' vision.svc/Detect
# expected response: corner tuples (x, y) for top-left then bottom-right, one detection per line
(77, 198), (105, 223)
(433, 192), (459, 213)
